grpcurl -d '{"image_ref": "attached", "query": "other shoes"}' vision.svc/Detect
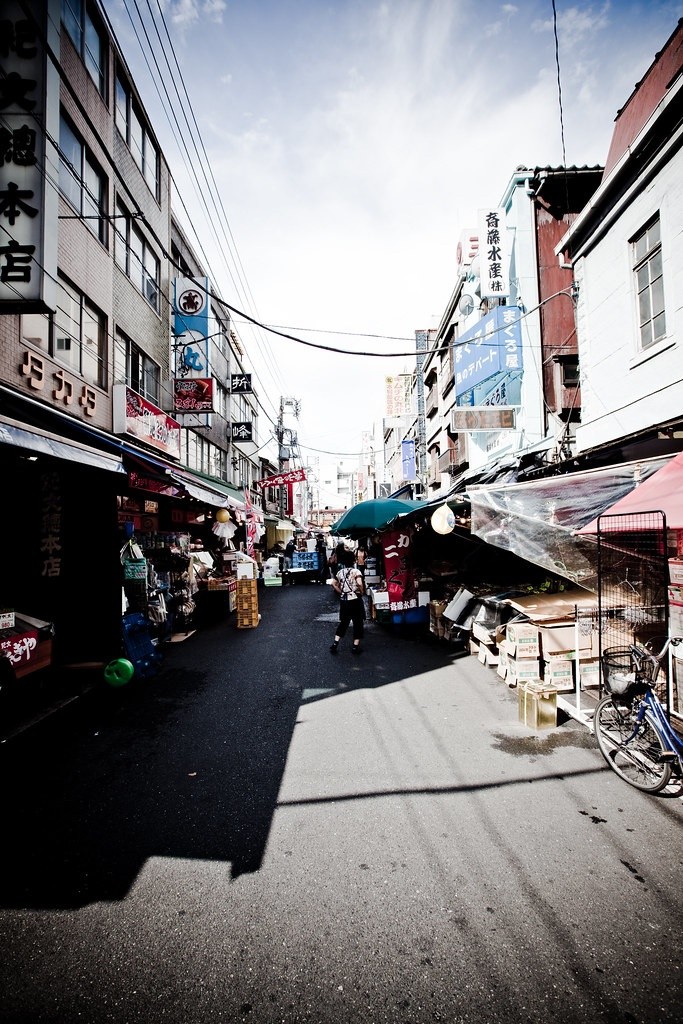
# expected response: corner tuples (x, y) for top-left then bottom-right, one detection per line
(330, 641), (338, 650)
(352, 647), (362, 654)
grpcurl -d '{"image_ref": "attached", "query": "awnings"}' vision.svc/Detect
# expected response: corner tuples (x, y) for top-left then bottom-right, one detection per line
(77, 427), (279, 523)
(400, 449), (683, 566)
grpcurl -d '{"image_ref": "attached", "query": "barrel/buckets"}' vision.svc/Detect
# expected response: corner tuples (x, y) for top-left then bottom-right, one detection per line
(104, 658), (134, 686)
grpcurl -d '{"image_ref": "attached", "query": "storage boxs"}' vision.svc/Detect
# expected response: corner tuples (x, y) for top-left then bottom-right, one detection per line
(121, 550), (604, 729)
(0, 610), (54, 679)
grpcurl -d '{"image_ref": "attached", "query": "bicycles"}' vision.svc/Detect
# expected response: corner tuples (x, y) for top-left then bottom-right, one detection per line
(593, 634), (682, 796)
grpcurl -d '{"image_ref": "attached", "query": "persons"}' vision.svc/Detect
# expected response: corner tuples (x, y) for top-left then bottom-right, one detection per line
(274, 537), (306, 586)
(353, 544), (368, 574)
(329, 551), (367, 654)
(313, 534), (354, 585)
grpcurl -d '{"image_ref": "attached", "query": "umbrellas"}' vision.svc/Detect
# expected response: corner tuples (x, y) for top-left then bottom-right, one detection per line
(329, 498), (428, 541)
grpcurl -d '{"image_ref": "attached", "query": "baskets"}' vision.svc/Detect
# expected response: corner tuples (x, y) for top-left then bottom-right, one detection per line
(602, 646), (653, 695)
